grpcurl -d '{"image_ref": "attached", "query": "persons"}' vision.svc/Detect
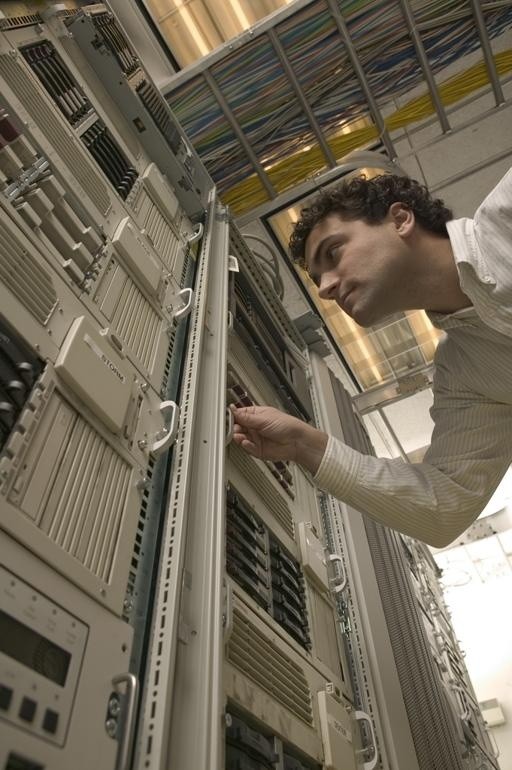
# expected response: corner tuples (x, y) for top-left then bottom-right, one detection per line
(226, 165), (512, 548)
